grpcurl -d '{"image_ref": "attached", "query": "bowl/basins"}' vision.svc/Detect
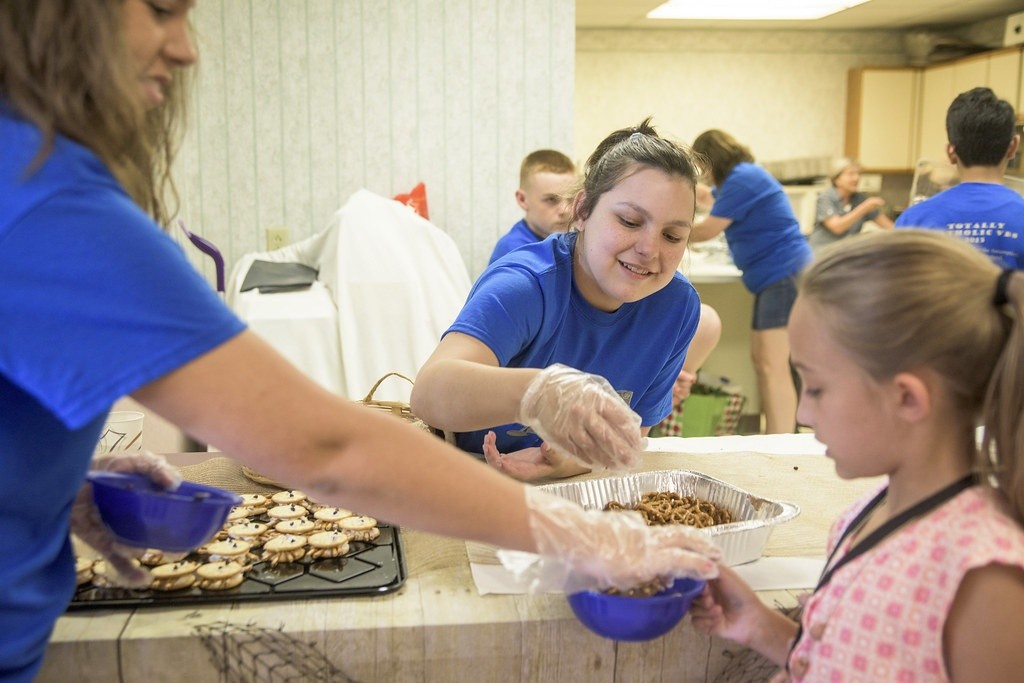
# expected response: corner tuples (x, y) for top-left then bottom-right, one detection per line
(569, 578), (708, 639)
(86, 470), (247, 552)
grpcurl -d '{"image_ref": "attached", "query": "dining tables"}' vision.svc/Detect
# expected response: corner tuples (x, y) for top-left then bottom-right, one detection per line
(32, 433), (890, 683)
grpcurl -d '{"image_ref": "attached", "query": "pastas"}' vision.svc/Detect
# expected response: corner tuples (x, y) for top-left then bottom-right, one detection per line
(602, 490), (735, 528)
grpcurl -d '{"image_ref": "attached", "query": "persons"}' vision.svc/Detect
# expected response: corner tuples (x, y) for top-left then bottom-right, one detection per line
(686, 129), (810, 435)
(409, 115), (704, 482)
(490, 149), (579, 263)
(894, 86), (1023, 269)
(689, 224), (1023, 682)
(1, 0), (717, 681)
(815, 157), (892, 244)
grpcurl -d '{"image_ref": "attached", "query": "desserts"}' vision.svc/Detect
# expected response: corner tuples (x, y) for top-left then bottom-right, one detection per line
(68, 489), (381, 591)
